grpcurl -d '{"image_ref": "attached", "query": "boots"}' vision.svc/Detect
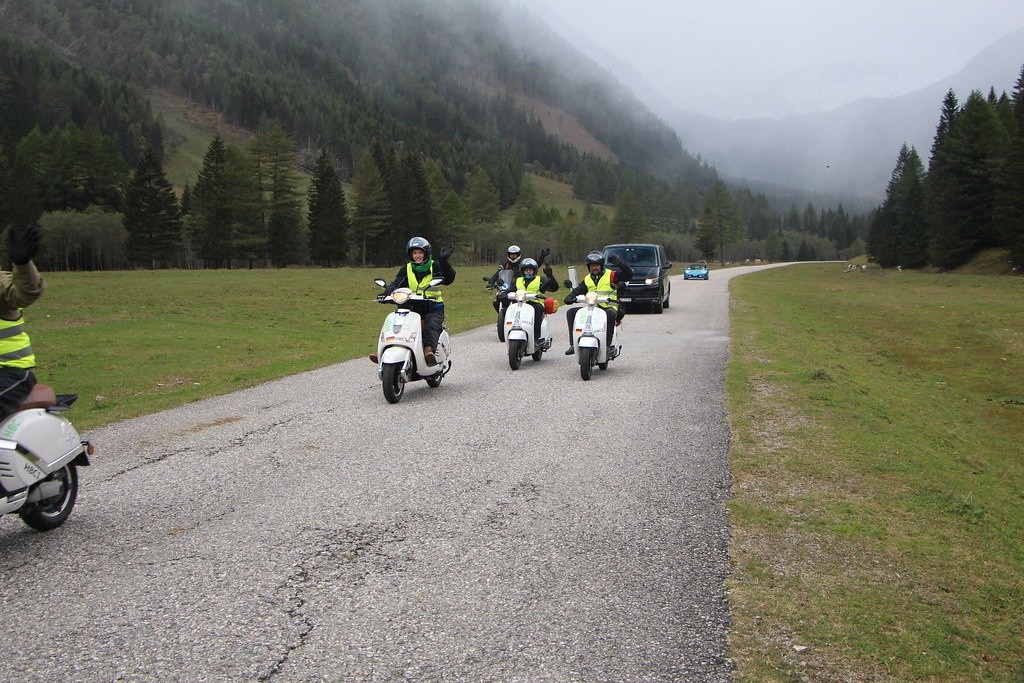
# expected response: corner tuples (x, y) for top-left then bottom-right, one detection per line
(369, 353), (378, 364)
(423, 346), (434, 364)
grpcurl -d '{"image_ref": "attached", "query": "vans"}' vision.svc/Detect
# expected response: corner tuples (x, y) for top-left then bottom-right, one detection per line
(600, 243), (673, 314)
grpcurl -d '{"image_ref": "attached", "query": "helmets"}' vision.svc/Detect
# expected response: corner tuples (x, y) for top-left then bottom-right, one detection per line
(507, 245), (521, 253)
(585, 251), (605, 265)
(406, 237), (432, 253)
(519, 257), (538, 272)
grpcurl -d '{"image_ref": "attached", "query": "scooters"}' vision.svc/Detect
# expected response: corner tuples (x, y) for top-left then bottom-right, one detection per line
(1, 383), (95, 532)
(481, 268), (519, 343)
(496, 277), (553, 370)
(562, 278), (626, 381)
(370, 278), (453, 404)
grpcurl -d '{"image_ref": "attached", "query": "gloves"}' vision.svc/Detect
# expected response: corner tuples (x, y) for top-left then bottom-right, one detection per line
(377, 293), (387, 304)
(0, 223), (44, 267)
(540, 248), (552, 258)
(607, 255), (621, 267)
(564, 295), (575, 305)
(438, 244), (456, 260)
(543, 261), (552, 278)
(497, 292), (507, 303)
(486, 283), (493, 290)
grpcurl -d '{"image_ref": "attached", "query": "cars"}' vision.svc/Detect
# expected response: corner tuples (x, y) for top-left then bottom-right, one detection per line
(683, 263), (710, 281)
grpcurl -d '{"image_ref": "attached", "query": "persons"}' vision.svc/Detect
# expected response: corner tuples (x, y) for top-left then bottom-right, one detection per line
(0, 223), (46, 419)
(486, 245), (559, 347)
(564, 251), (634, 355)
(370, 237), (456, 364)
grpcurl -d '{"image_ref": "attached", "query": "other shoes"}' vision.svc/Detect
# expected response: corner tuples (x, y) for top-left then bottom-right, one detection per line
(565, 345), (574, 355)
(534, 338), (538, 347)
